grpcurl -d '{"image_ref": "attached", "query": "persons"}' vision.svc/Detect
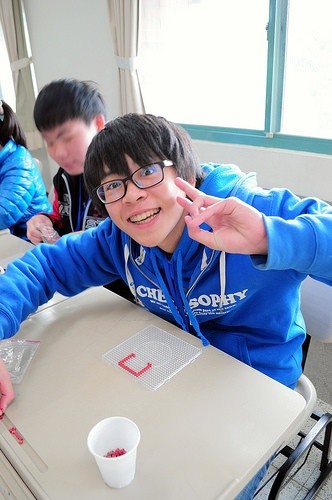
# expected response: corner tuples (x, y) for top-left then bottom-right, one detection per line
(0, 113), (332, 500)
(0, 99), (54, 245)
(27, 79), (136, 303)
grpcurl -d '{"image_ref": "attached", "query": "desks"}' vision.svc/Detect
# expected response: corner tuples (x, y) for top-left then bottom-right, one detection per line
(0, 231), (70, 315)
(0, 285), (307, 500)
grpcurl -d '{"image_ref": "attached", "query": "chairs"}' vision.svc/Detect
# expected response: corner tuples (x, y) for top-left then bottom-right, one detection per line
(253, 274), (332, 500)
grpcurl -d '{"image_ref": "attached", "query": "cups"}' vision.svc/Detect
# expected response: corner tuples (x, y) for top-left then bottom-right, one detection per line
(87, 416), (141, 489)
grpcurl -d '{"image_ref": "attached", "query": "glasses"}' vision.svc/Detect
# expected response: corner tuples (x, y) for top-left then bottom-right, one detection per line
(92, 158), (174, 204)
